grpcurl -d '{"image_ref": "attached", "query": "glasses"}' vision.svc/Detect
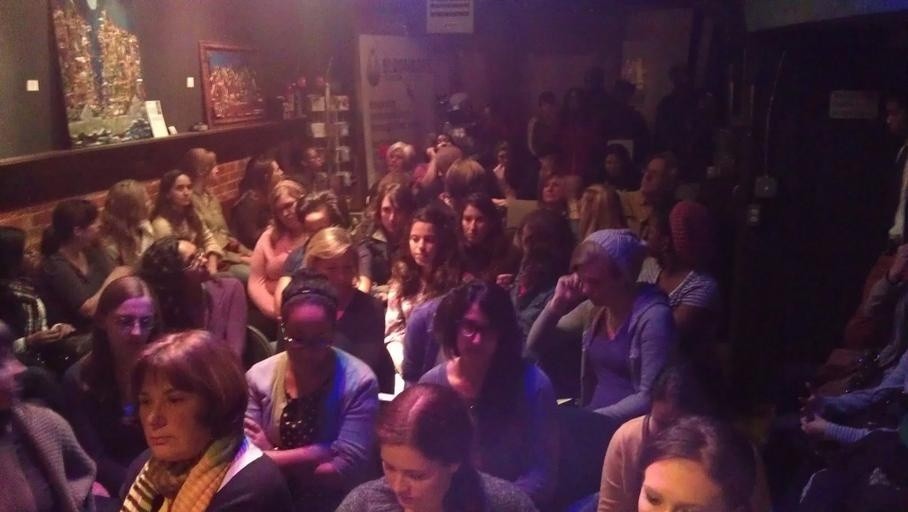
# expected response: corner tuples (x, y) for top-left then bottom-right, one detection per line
(179, 247), (206, 272)
(456, 319), (507, 340)
(101, 313), (155, 331)
(278, 320), (333, 351)
(273, 195), (302, 214)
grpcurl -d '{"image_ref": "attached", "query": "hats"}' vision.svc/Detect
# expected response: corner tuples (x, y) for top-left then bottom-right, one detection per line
(580, 226), (640, 274)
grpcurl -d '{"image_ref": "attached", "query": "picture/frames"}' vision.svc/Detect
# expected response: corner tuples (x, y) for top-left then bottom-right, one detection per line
(198, 38), (269, 128)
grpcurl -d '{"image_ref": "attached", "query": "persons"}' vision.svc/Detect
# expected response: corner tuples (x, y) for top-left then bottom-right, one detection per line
(2, 62), (907, 510)
